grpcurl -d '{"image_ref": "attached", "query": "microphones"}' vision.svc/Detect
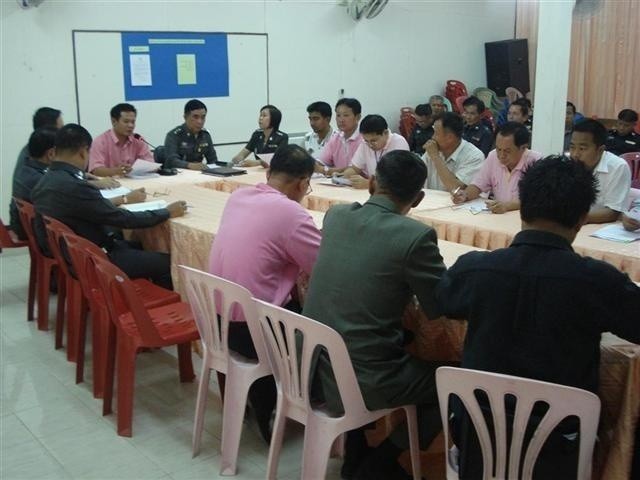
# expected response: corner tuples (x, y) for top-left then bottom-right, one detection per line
(133, 132), (160, 152)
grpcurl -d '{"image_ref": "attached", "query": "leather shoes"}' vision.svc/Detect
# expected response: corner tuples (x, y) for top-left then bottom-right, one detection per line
(340, 460), (425, 480)
(248, 399), (271, 444)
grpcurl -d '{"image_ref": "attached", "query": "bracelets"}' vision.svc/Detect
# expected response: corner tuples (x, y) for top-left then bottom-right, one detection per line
(122, 195), (128, 204)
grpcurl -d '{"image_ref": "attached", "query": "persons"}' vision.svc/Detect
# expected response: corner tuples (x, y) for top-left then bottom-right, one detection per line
(232, 96), (409, 189)
(563, 99), (639, 231)
(440, 155), (640, 480)
(10, 127), (120, 241)
(29, 124), (188, 291)
(86, 104), (155, 177)
(17, 107), (63, 159)
(162, 100), (218, 171)
(408, 96), (539, 215)
(206, 144), (322, 447)
(297, 150), (447, 480)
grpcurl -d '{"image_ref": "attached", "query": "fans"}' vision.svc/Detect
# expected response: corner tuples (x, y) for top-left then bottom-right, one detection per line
(18, 0), (44, 11)
(573, 0), (606, 20)
(342, 0), (389, 21)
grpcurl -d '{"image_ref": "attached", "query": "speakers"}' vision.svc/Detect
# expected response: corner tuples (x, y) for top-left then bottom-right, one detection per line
(484, 38), (529, 97)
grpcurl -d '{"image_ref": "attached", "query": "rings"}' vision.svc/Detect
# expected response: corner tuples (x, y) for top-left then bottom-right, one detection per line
(122, 170), (127, 174)
(120, 166), (125, 170)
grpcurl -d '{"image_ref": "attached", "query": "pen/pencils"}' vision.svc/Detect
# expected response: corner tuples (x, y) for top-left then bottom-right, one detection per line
(453, 186), (461, 196)
(128, 188), (152, 196)
(184, 205), (195, 208)
(326, 175), (345, 178)
